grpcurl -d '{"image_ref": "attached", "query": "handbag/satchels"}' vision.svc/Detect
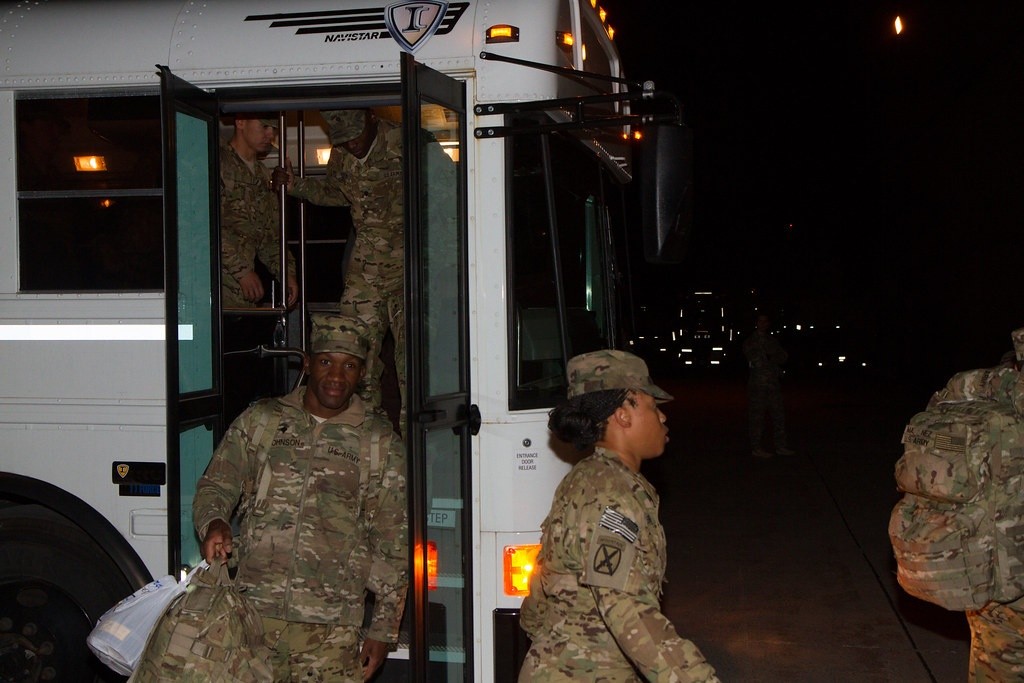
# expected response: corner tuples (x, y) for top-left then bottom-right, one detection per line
(86, 559), (211, 677)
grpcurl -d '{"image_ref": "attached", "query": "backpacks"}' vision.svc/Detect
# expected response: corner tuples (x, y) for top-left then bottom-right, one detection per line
(125, 556), (274, 683)
(888, 370), (1024, 611)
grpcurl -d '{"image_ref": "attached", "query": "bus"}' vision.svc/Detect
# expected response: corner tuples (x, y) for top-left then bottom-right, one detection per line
(1, 0), (691, 682)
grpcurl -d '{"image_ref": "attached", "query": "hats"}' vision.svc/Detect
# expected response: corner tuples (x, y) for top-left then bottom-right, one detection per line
(1012, 327), (1024, 361)
(310, 312), (370, 360)
(566, 350), (674, 404)
(260, 118), (279, 128)
(320, 107), (372, 146)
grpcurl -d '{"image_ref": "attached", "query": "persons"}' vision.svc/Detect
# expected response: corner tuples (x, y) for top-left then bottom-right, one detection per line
(272, 108), (405, 441)
(193, 315), (409, 683)
(962, 327), (1024, 683)
(730, 314), (802, 459)
(517, 349), (722, 683)
(220, 112), (301, 310)
